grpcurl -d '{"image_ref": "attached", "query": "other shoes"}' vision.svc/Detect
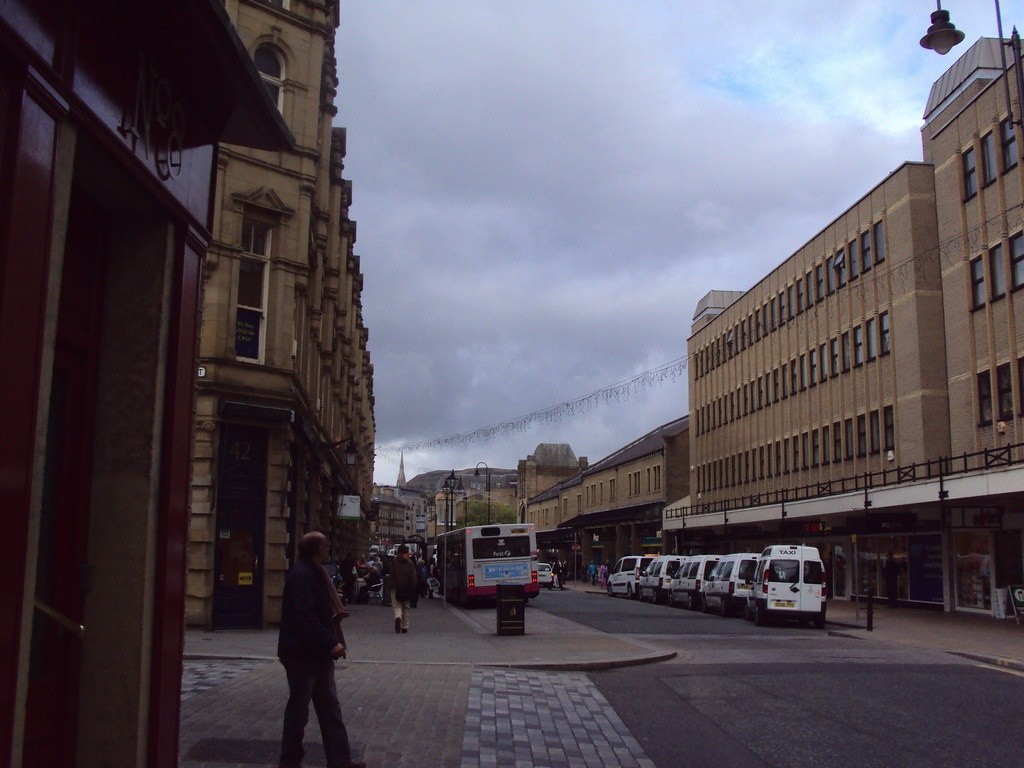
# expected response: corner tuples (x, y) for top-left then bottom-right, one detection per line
(394, 617), (401, 633)
(349, 761), (366, 768)
(402, 629), (407, 633)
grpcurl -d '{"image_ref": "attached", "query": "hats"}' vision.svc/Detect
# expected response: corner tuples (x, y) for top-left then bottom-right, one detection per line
(397, 544), (409, 552)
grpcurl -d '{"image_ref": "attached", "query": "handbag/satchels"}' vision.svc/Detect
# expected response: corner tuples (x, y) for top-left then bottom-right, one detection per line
(426, 576), (441, 590)
(395, 584), (416, 602)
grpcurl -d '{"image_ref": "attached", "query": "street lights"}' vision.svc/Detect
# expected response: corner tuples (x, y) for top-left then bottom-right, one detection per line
(442, 480), (451, 531)
(474, 461), (491, 524)
(445, 469), (460, 530)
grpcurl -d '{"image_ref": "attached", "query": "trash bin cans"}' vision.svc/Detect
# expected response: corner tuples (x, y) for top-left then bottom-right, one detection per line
(994, 583), (1009, 620)
(1008, 584), (1024, 621)
(495, 582), (526, 636)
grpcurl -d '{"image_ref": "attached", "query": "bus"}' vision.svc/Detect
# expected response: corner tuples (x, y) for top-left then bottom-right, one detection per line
(438, 524), (541, 607)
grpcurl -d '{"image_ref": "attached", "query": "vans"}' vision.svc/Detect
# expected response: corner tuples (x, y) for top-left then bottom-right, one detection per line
(667, 554), (724, 611)
(607, 556), (653, 600)
(746, 545), (827, 627)
(638, 555), (690, 605)
(703, 552), (762, 619)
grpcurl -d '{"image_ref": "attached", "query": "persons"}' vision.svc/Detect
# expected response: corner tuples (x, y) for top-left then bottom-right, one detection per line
(553, 558), (569, 591)
(276, 532), (365, 768)
(581, 559), (588, 584)
(588, 561), (598, 586)
(389, 544), (418, 633)
(356, 550), (389, 599)
(599, 560), (609, 590)
(428, 558), (439, 598)
(410, 547), (437, 597)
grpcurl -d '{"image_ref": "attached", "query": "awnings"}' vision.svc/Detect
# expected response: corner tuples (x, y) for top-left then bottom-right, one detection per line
(556, 500), (663, 529)
(535, 528), (575, 541)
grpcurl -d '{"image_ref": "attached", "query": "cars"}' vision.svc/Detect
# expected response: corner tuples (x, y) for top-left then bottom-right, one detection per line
(537, 562), (555, 590)
